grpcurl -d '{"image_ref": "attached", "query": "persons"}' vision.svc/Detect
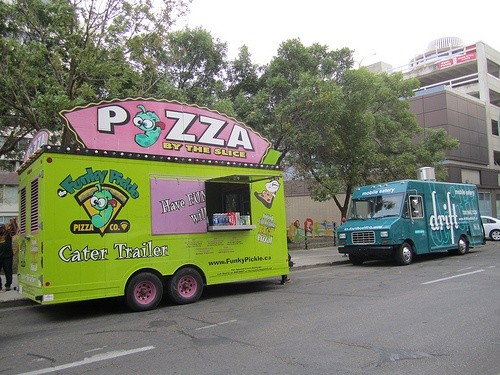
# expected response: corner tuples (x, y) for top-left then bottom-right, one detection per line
(0, 216), (19, 291)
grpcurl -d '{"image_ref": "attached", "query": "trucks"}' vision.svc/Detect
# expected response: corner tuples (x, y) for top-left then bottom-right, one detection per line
(337, 166), (488, 265)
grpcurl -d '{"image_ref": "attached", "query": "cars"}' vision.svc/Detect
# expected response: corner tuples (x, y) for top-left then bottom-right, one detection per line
(480, 216), (500, 240)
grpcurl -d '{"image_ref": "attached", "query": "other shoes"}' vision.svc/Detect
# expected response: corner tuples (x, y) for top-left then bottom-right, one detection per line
(6, 287), (10, 290)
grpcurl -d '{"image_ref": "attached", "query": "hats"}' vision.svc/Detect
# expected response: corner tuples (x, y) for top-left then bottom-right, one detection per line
(0, 223), (5, 228)
